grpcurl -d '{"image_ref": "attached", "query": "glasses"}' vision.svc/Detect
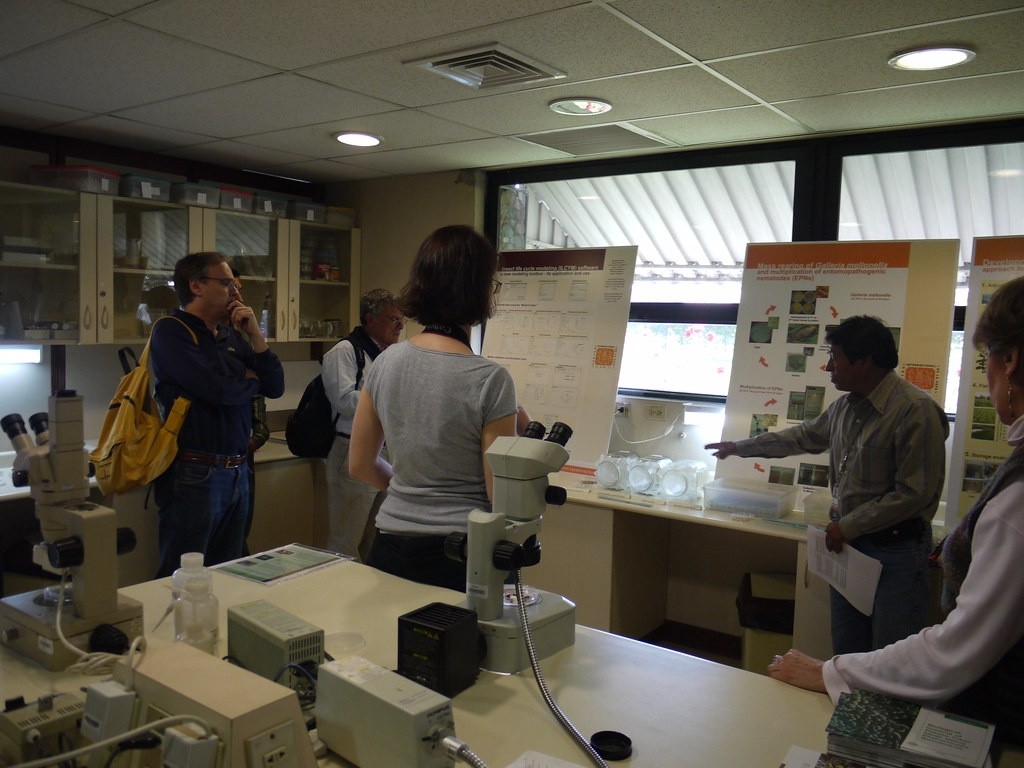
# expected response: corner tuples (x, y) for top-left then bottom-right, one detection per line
(829, 350), (844, 361)
(371, 312), (408, 327)
(200, 275), (236, 287)
(491, 278), (502, 294)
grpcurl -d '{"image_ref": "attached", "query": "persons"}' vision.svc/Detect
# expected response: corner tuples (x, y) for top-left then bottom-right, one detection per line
(702, 314), (948, 658)
(769, 274), (1024, 751)
(149, 252), (285, 578)
(319, 288), (406, 565)
(346, 227), (534, 592)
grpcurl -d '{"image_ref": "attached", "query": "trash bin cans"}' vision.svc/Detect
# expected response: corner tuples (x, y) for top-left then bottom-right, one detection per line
(738, 568), (795, 672)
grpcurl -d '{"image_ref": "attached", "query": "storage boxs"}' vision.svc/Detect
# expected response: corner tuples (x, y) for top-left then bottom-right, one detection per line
(24, 329), (50, 339)
(736, 572), (795, 635)
(253, 193), (287, 217)
(327, 206), (357, 227)
(287, 199), (326, 223)
(701, 476), (799, 519)
(120, 173), (171, 202)
(741, 628), (794, 677)
(219, 186), (254, 213)
(35, 319), (78, 340)
(3, 237), (52, 265)
(804, 490), (831, 525)
(170, 182), (220, 209)
(29, 165), (121, 196)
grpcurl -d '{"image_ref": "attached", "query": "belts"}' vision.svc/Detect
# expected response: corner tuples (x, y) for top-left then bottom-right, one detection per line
(336, 432), (386, 447)
(912, 521), (931, 534)
(176, 452), (248, 468)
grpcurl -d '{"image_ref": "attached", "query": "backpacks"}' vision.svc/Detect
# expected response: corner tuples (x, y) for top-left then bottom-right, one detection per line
(285, 336), (366, 459)
(88, 315), (199, 497)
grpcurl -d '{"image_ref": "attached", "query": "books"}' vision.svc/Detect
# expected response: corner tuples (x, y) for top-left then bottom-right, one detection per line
(777, 689), (995, 768)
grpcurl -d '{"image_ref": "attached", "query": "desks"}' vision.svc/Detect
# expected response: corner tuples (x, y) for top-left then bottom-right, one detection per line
(0, 542), (1024, 767)
(520, 473), (832, 675)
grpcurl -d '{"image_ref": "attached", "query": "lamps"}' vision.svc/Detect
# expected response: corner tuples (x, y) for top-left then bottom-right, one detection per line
(0, 344), (44, 364)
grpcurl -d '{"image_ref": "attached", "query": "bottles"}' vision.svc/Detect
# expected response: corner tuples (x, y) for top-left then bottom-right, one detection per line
(173, 553), (212, 607)
(176, 617), (215, 657)
(596, 450), (640, 488)
(660, 459), (709, 502)
(627, 455), (673, 493)
(175, 576), (220, 653)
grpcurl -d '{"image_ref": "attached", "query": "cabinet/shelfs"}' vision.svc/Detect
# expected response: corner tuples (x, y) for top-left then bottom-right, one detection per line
(0, 180), (361, 347)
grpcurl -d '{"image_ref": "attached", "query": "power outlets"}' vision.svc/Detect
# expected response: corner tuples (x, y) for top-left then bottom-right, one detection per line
(614, 401), (630, 417)
(645, 403), (668, 420)
